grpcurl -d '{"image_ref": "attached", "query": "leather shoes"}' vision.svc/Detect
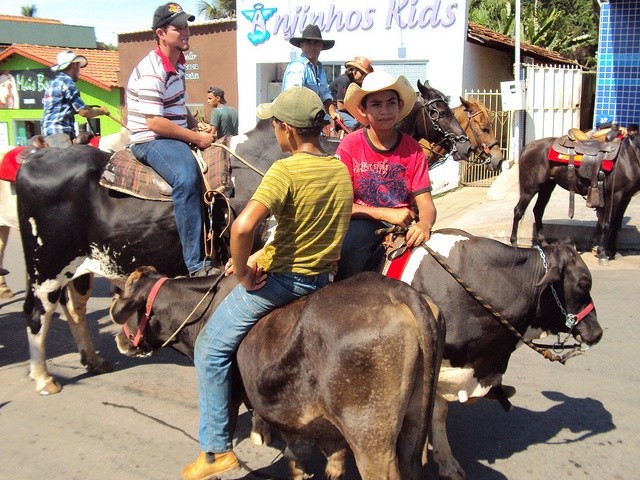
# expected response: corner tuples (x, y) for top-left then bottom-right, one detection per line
(180, 451), (239, 479)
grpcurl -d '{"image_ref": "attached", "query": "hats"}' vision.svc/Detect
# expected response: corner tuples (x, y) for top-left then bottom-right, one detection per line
(345, 57), (373, 74)
(255, 86), (326, 127)
(344, 71), (416, 126)
(289, 25), (334, 50)
(51, 50), (88, 70)
(152, 3), (194, 27)
(210, 88), (226, 104)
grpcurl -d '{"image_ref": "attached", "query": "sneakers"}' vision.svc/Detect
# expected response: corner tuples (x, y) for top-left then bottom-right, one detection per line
(191, 263), (221, 277)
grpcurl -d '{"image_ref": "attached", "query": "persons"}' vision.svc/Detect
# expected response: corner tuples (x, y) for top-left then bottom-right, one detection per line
(280, 24), (342, 128)
(36, 50), (111, 148)
(207, 87), (237, 137)
(334, 72), (437, 280)
(0, 72), (19, 109)
(180, 86), (356, 480)
(126, 1), (222, 279)
(323, 55), (373, 130)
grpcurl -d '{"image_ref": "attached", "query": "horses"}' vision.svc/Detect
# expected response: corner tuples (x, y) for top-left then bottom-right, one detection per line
(0, 126), (132, 301)
(418, 98), (503, 174)
(508, 130), (640, 266)
(247, 80), (473, 161)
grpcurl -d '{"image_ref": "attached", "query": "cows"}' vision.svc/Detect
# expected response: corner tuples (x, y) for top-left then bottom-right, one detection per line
(15, 118), (292, 395)
(109, 265), (446, 480)
(379, 228), (602, 480)
